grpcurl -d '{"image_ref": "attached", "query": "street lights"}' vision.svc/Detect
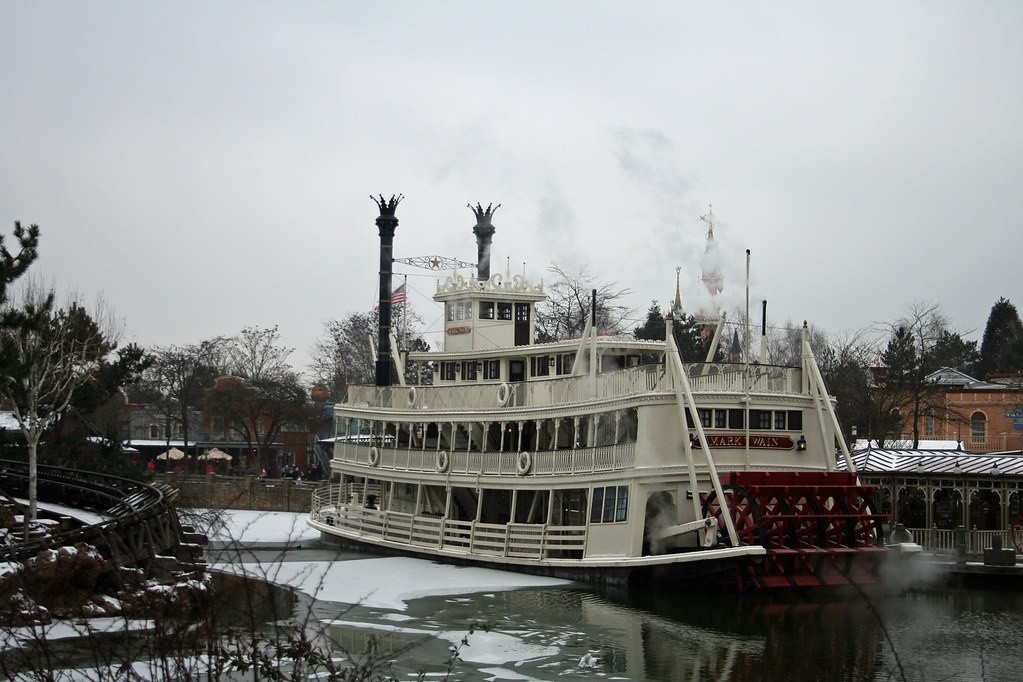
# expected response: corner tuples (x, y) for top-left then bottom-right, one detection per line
(202, 446), (210, 475)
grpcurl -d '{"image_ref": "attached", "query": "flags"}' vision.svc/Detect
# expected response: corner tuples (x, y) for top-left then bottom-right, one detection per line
(391, 282), (406, 304)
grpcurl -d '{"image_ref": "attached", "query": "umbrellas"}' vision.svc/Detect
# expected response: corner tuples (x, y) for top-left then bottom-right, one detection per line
(156, 447), (192, 467)
(198, 447), (233, 465)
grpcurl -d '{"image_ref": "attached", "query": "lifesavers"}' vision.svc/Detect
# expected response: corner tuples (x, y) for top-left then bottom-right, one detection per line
(407, 387), (417, 408)
(367, 447), (378, 466)
(889, 528), (914, 545)
(518, 451), (531, 475)
(436, 451), (448, 473)
(496, 383), (509, 406)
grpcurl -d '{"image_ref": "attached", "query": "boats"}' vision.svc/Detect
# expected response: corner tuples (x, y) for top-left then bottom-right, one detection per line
(305, 190), (925, 607)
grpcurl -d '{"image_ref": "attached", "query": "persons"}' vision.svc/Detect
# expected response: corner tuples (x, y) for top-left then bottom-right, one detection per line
(984, 508), (996, 530)
(279, 463), (318, 481)
(146, 457), (227, 475)
